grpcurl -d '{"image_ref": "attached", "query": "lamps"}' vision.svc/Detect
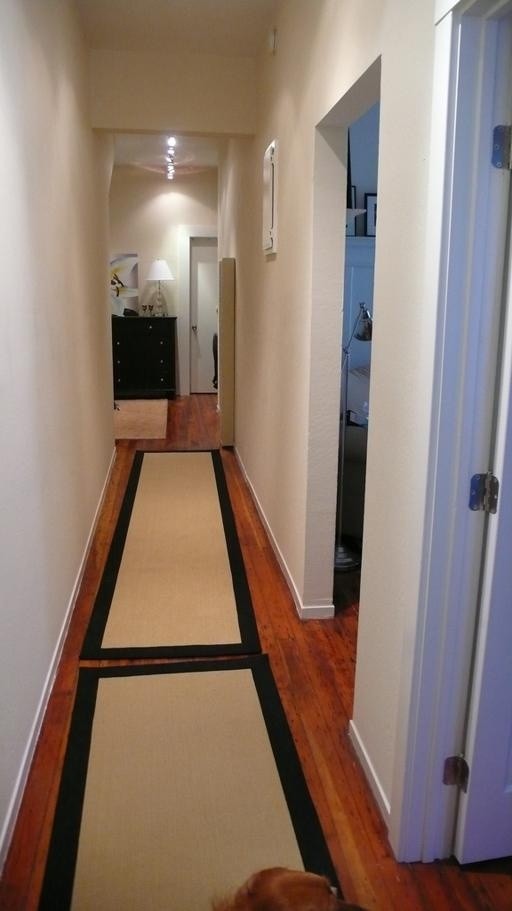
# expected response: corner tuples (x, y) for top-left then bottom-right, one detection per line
(335, 302), (371, 570)
(164, 136), (177, 180)
(145, 259), (174, 317)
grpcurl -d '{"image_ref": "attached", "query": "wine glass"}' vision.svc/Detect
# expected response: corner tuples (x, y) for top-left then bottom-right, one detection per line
(141, 304), (153, 317)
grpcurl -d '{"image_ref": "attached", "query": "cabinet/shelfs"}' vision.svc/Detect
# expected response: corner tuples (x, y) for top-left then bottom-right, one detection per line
(340, 236), (375, 427)
(112, 316), (177, 401)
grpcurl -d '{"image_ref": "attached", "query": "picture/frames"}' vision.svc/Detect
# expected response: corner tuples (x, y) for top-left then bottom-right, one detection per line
(363, 193), (377, 236)
(346, 186), (357, 237)
(261, 139), (279, 257)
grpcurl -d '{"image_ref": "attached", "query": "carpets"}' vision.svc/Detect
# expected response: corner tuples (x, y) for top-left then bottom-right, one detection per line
(114, 398), (168, 440)
(36, 656), (343, 911)
(78, 449), (262, 661)
